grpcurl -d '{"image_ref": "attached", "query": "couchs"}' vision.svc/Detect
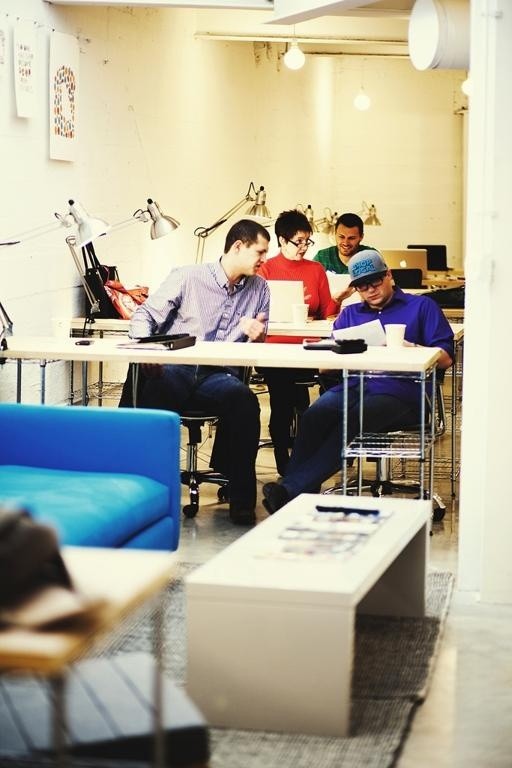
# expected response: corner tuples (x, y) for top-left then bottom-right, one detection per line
(0, 402), (179, 558)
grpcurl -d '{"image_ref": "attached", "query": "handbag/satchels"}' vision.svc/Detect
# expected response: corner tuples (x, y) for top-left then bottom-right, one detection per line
(86, 265), (149, 319)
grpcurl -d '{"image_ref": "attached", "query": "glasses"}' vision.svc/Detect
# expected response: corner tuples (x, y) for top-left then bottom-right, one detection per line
(355, 277), (384, 292)
(286, 238), (315, 248)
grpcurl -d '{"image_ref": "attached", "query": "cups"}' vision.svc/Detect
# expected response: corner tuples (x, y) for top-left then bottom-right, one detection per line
(292, 304), (310, 327)
(384, 324), (407, 352)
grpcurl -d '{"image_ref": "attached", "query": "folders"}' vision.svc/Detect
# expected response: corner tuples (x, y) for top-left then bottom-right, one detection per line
(117, 337), (195, 350)
(133, 333), (189, 343)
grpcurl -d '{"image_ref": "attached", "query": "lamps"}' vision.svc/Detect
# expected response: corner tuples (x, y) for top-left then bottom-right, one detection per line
(65, 198), (179, 337)
(0, 198), (113, 250)
(193, 182), (382, 266)
(285, 24), (305, 69)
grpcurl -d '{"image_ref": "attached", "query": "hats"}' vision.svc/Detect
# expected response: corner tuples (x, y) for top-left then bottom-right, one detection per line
(348, 249), (387, 288)
(1, 507), (103, 626)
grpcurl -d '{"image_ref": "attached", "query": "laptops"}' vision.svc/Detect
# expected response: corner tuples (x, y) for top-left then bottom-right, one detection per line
(382, 249), (433, 279)
(406, 245), (454, 271)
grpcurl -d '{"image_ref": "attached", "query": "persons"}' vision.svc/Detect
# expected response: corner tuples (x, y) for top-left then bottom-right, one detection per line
(115, 219), (272, 526)
(260, 248), (458, 516)
(310, 211), (380, 275)
(250, 208), (354, 485)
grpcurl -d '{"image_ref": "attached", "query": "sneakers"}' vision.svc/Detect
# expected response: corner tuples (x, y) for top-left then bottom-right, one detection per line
(230, 503), (255, 525)
(262, 483), (286, 514)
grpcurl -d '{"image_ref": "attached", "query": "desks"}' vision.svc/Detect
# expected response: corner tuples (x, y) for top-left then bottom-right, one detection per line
(0, 542), (174, 767)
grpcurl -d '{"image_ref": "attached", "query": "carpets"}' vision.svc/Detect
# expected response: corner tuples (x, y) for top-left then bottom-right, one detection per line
(83, 560), (453, 768)
